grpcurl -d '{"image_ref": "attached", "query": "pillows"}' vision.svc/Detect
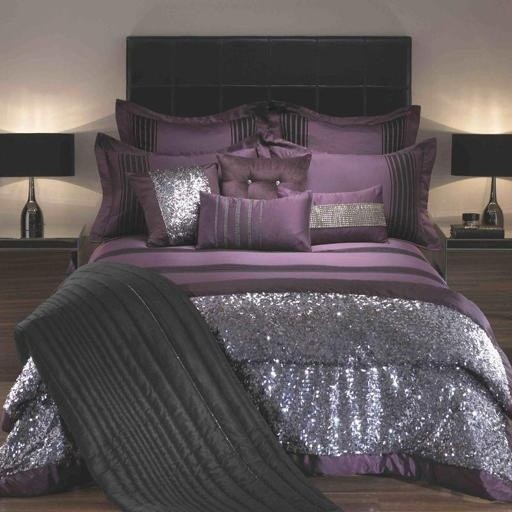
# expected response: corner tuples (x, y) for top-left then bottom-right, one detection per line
(89, 131), (271, 245)
(196, 192), (314, 250)
(123, 164), (224, 245)
(267, 102), (421, 156)
(110, 96), (271, 156)
(274, 185), (390, 247)
(258, 134), (446, 248)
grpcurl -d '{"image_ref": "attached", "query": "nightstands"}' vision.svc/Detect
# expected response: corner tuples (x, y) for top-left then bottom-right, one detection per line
(433, 216), (512, 356)
(0, 235), (77, 381)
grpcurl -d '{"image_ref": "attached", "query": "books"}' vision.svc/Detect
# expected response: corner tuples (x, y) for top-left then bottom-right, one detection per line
(448, 223), (504, 240)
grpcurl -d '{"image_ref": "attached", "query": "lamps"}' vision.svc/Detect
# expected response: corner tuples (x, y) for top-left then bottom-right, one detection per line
(1, 133), (76, 239)
(451, 135), (511, 234)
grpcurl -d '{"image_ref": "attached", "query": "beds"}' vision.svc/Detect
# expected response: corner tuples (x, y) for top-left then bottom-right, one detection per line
(1, 33), (510, 512)
